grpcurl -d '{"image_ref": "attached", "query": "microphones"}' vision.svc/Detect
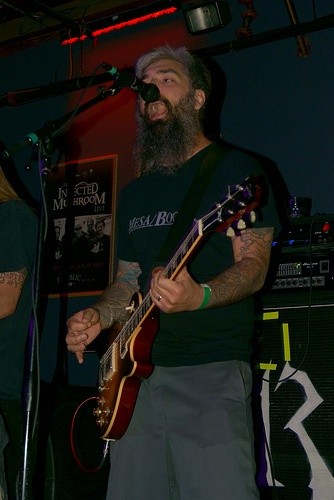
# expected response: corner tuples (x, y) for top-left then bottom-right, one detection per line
(104, 63), (160, 103)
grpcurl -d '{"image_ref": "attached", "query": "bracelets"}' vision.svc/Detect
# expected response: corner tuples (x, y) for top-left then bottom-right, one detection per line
(196, 283), (214, 310)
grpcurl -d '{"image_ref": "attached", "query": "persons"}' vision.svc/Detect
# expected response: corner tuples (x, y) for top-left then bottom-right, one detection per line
(59, 42), (278, 500)
(0, 161), (42, 500)
(55, 217), (110, 268)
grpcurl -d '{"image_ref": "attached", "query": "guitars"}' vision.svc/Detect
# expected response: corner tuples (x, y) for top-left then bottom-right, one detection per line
(89, 169), (266, 442)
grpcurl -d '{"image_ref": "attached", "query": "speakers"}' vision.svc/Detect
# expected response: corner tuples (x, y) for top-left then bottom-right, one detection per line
(252, 292), (334, 494)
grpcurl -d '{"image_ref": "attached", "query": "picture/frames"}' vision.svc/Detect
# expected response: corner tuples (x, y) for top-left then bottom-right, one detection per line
(39, 153), (119, 297)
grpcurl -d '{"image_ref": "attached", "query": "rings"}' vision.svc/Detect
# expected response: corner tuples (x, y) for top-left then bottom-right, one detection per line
(157, 295), (163, 302)
